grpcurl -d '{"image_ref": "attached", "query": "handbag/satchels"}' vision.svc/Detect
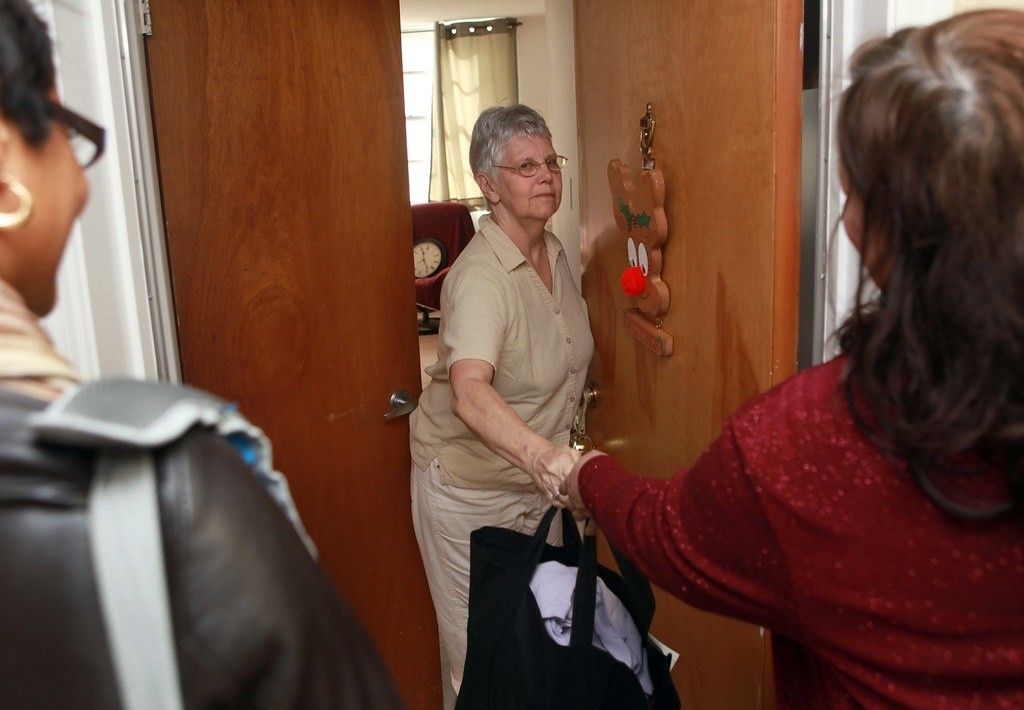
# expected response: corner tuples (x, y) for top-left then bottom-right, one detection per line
(454, 507), (684, 710)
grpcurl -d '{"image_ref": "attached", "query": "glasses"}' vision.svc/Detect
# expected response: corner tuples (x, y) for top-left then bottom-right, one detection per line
(494, 156), (568, 177)
(41, 98), (107, 169)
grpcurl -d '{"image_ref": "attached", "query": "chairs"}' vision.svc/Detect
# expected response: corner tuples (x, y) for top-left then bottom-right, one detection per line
(411, 202), (477, 335)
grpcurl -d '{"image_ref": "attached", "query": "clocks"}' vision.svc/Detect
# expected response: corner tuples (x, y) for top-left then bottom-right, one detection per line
(412, 239), (447, 278)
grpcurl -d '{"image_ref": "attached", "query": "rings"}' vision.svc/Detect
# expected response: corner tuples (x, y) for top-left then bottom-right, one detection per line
(553, 492), (560, 501)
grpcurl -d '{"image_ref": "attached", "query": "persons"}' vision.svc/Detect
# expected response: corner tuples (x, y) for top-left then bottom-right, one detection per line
(557, 4), (1023, 708)
(0, 0), (403, 710)
(410, 100), (600, 710)
